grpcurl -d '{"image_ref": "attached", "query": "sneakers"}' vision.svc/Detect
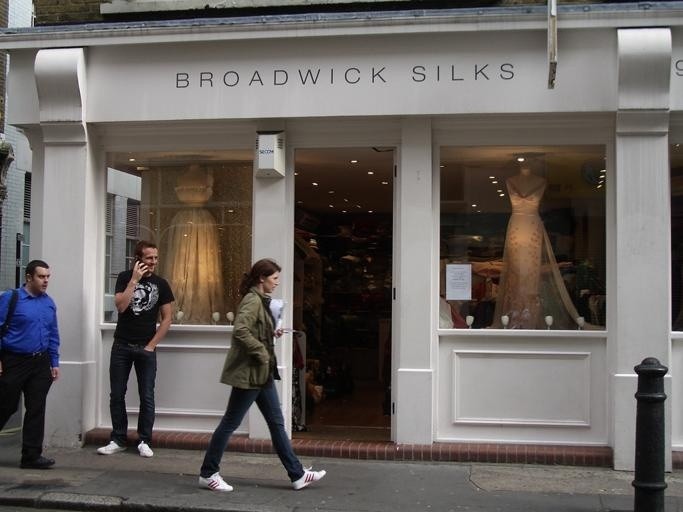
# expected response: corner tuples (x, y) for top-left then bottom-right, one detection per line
(96, 439), (129, 455)
(198, 472), (235, 493)
(137, 440), (155, 458)
(292, 468), (327, 490)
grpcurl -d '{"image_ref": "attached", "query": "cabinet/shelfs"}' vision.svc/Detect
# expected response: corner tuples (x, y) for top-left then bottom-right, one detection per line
(293, 233), (392, 349)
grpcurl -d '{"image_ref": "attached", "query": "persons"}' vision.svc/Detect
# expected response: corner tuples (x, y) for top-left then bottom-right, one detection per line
(96, 238), (175, 460)
(198, 257), (327, 492)
(487, 166), (601, 331)
(158, 163), (228, 324)
(1, 258), (60, 470)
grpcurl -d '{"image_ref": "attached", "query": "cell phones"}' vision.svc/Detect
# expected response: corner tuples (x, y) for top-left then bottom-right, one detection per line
(135, 257), (148, 274)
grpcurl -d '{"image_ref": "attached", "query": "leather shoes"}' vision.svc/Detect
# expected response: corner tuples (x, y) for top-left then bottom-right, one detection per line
(21, 456), (57, 469)
